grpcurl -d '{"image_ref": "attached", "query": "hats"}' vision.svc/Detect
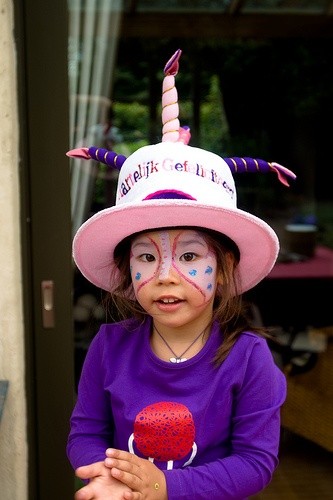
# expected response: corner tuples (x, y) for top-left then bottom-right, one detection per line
(71, 141), (280, 302)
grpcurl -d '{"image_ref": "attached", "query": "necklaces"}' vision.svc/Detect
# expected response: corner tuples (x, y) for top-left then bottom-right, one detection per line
(152, 322), (205, 363)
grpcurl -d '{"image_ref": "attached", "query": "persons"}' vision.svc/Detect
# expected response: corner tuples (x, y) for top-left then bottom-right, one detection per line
(67, 142), (287, 500)
